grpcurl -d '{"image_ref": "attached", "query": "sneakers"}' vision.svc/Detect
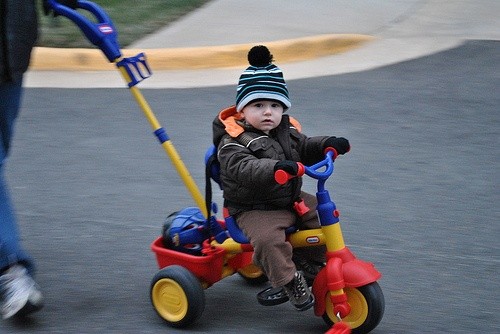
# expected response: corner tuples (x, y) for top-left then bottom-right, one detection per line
(297, 260), (326, 281)
(0, 265), (45, 323)
(284, 274), (315, 313)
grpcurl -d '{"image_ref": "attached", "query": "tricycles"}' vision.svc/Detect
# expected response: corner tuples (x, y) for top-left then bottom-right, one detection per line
(46, 0), (386, 334)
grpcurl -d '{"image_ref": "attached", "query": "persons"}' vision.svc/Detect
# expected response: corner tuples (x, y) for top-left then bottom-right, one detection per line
(0, 0), (76, 319)
(215, 63), (351, 312)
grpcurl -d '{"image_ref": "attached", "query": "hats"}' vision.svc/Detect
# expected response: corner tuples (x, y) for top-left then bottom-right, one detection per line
(236, 45), (291, 112)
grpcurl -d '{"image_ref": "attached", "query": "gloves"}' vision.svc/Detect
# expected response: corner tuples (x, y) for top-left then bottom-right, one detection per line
(324, 136), (350, 155)
(274, 160), (298, 175)
(42, 0), (78, 17)
